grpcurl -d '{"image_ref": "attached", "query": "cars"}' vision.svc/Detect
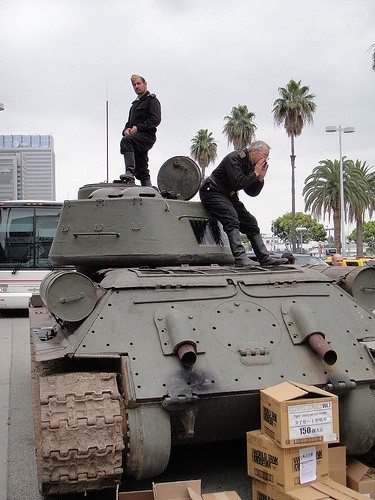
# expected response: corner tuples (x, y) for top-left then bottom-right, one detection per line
(272, 254), (329, 269)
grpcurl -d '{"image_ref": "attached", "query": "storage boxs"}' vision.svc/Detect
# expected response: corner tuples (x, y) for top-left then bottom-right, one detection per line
(245, 429), (331, 489)
(258, 381), (342, 447)
(347, 459), (375, 495)
(248, 478), (370, 500)
(183, 486), (242, 499)
(113, 477), (201, 499)
(327, 444), (351, 486)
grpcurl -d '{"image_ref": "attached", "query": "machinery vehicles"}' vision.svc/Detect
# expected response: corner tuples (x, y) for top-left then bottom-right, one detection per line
(28, 155), (374, 495)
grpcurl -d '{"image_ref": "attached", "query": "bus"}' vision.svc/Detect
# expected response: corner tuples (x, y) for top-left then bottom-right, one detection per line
(324, 248), (337, 260)
(0, 200), (74, 309)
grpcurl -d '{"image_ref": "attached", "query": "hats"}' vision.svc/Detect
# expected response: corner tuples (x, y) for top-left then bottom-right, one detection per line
(283, 253), (298, 260)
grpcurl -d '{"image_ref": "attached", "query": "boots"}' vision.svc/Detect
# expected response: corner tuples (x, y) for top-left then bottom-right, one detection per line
(226, 229), (260, 267)
(141, 180), (152, 187)
(120, 152), (135, 182)
(247, 234), (289, 266)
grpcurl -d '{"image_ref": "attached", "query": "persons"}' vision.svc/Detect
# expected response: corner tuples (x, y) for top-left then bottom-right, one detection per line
(120, 74), (162, 187)
(198, 140), (289, 268)
(317, 240), (323, 257)
(331, 253), (344, 266)
(281, 252), (295, 265)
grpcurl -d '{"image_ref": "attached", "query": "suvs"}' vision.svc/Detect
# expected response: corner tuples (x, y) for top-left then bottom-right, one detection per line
(324, 256), (374, 266)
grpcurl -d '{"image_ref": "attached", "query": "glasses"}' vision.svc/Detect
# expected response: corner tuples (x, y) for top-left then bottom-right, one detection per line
(257, 149), (270, 161)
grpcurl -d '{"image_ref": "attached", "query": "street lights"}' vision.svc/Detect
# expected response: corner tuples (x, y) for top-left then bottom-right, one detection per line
(325, 124), (356, 255)
